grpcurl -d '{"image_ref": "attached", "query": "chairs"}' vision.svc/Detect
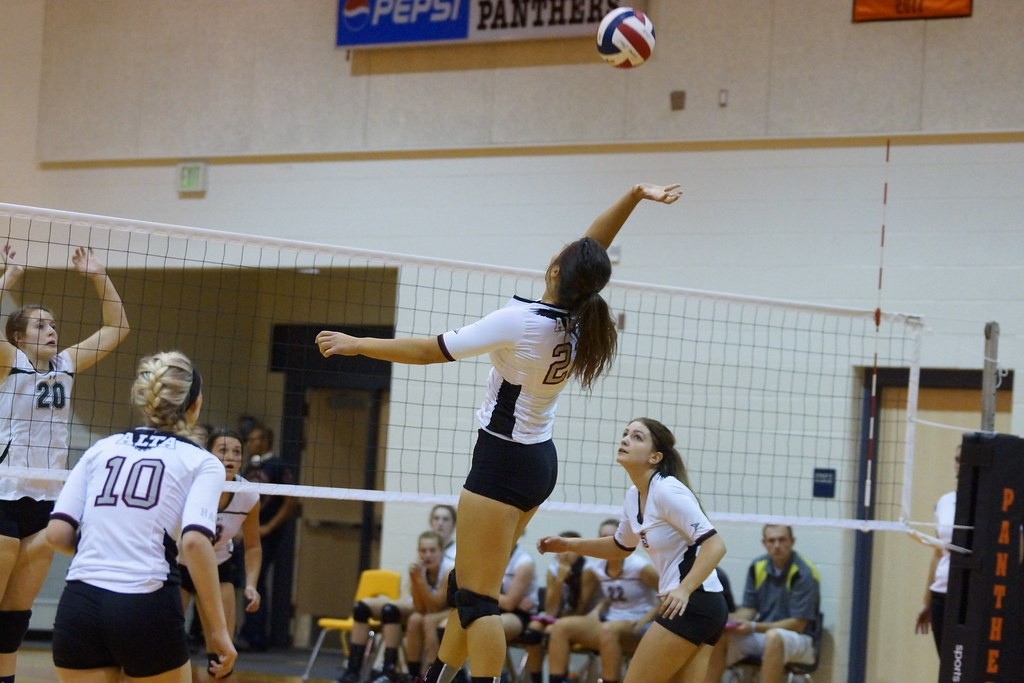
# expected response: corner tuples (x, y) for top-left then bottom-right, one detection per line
(302, 570), (824, 683)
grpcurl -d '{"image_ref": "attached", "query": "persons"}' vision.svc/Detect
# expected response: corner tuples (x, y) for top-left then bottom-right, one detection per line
(40, 351), (240, 683)
(912, 443), (962, 682)
(196, 412), (300, 640)
(536, 416), (730, 683)
(0, 242), (130, 682)
(338, 504), (824, 681)
(312, 180), (687, 681)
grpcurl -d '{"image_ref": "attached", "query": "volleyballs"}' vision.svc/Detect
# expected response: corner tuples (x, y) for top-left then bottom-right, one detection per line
(595, 4), (657, 71)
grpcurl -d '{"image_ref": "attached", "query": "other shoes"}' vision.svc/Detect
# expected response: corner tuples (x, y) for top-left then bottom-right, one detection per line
(233, 638), (269, 652)
(338, 671), (359, 683)
(369, 672), (393, 683)
